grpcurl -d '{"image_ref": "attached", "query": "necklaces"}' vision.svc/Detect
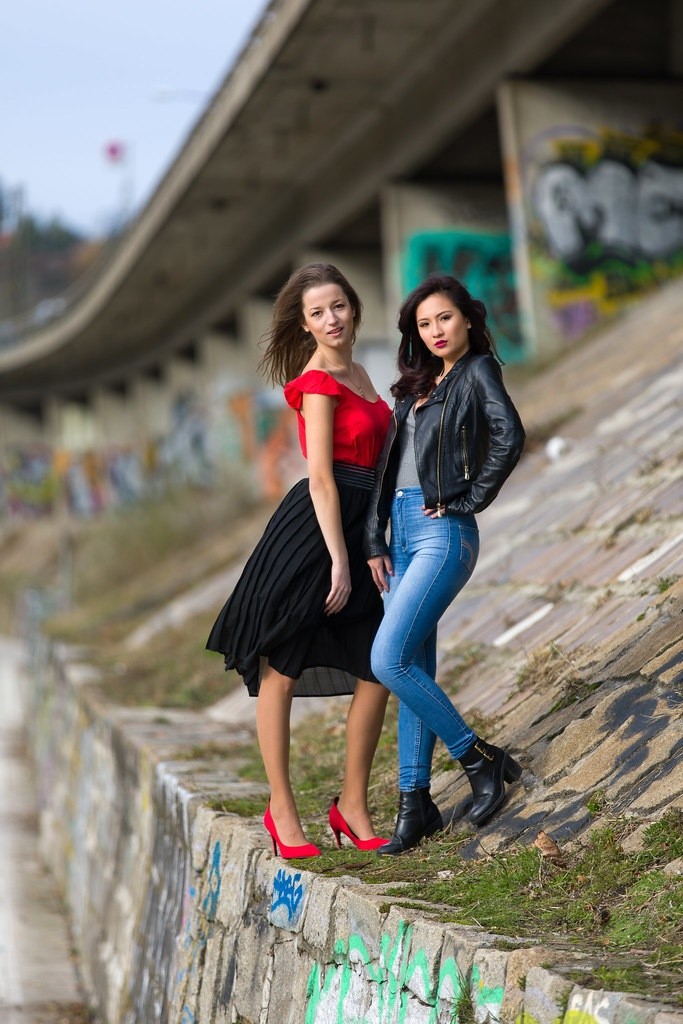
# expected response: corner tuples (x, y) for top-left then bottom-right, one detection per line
(315, 349), (365, 397)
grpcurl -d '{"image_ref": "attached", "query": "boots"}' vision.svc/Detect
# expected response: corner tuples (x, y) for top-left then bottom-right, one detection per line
(457, 734), (522, 824)
(377, 786), (444, 855)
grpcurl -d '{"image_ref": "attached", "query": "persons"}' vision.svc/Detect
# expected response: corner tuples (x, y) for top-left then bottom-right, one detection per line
(204, 268), (391, 859)
(365, 278), (527, 848)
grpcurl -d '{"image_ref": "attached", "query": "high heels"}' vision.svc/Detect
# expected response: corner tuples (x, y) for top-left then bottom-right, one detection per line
(264, 797), (321, 859)
(328, 796), (391, 851)
